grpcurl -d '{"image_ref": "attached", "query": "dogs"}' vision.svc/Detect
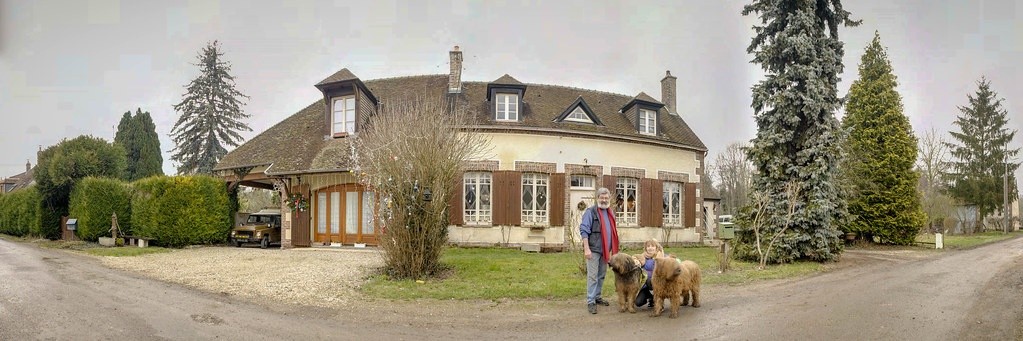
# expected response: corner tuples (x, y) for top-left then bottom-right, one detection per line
(647, 254), (702, 318)
(606, 252), (646, 314)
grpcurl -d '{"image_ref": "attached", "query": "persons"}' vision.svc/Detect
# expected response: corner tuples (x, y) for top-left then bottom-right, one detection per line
(633, 239), (665, 310)
(579, 186), (620, 314)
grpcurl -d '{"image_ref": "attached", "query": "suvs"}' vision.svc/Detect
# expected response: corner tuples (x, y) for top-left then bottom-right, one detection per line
(230, 212), (281, 249)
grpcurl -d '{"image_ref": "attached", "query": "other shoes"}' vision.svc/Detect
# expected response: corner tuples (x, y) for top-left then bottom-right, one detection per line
(588, 302), (597, 314)
(596, 297), (609, 306)
(647, 299), (654, 307)
